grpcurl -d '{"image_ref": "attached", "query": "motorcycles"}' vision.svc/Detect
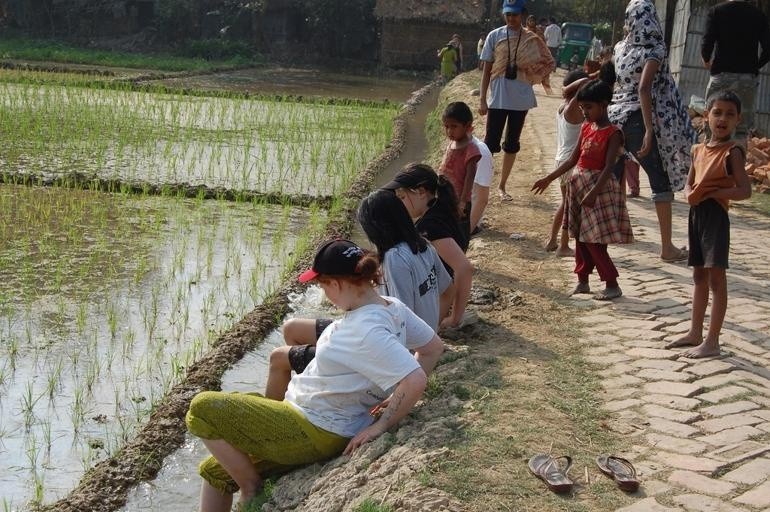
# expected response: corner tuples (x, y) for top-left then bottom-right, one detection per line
(557, 22), (597, 72)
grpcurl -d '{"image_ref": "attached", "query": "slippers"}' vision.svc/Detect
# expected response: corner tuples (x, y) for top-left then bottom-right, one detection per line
(595, 456), (638, 490)
(528, 453), (573, 492)
(662, 247), (689, 262)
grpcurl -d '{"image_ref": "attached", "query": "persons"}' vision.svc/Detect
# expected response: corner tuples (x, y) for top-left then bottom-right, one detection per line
(477, 1), (562, 201)
(700, 0), (770, 143)
(186, 239), (445, 512)
(437, 35), (463, 84)
(546, 70), (589, 257)
(264, 190), (456, 401)
(382, 163), (468, 281)
(531, 78), (636, 300)
(438, 102), (494, 235)
(607, 1), (690, 261)
(665, 90), (751, 359)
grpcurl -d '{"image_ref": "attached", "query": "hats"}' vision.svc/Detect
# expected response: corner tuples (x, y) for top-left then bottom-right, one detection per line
(299, 238), (365, 283)
(381, 172), (428, 190)
(502, 0), (525, 13)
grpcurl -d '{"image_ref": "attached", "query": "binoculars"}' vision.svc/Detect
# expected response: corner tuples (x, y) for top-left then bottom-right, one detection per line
(505, 62), (517, 79)
